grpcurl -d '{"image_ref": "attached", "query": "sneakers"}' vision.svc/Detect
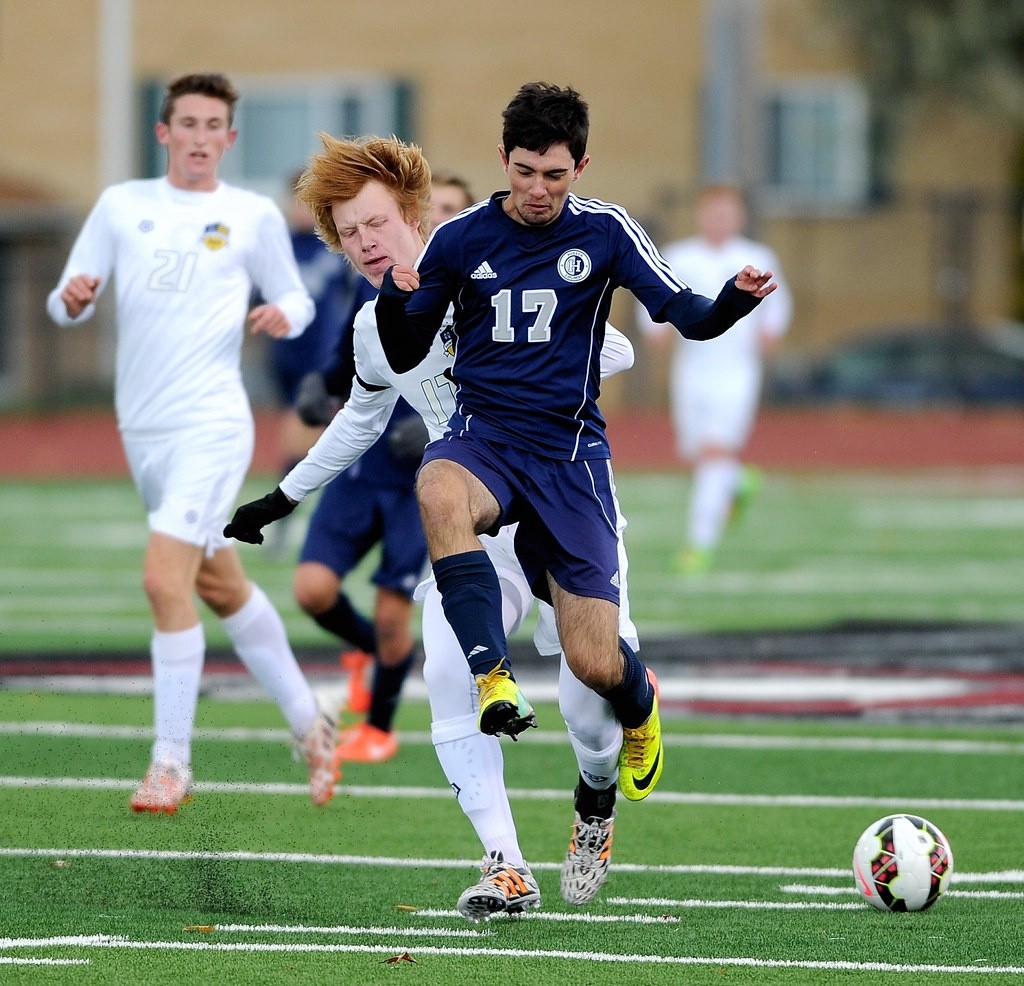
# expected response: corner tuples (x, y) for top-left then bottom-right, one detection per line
(617, 666), (665, 803)
(131, 765), (194, 813)
(340, 649), (373, 713)
(475, 655), (539, 742)
(297, 713), (343, 807)
(559, 784), (617, 906)
(456, 855), (541, 917)
(336, 722), (398, 763)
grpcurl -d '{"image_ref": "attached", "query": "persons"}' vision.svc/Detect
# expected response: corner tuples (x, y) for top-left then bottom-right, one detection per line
(222, 132), (640, 917)
(47, 74), (338, 814)
(641, 183), (797, 584)
(269, 168), (377, 484)
(376, 82), (778, 803)
(295, 176), (475, 762)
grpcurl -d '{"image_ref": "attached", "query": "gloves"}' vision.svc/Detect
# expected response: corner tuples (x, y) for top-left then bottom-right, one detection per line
(222, 486), (299, 544)
(389, 414), (430, 455)
(298, 372), (345, 430)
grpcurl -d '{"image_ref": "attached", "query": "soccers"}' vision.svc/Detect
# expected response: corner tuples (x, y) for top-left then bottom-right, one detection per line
(852, 813), (955, 912)
